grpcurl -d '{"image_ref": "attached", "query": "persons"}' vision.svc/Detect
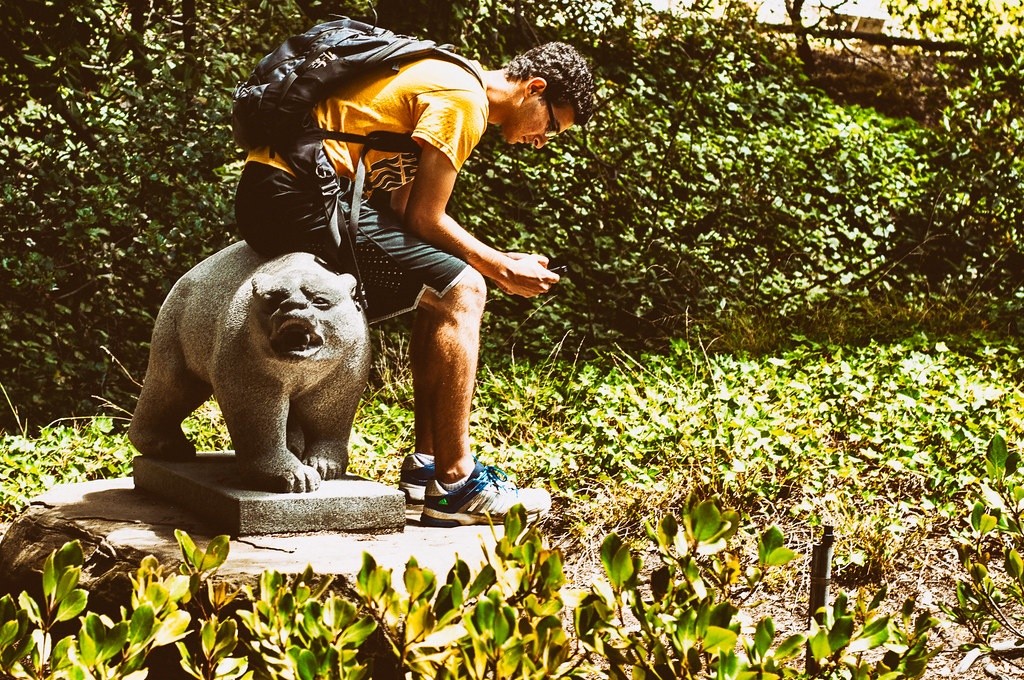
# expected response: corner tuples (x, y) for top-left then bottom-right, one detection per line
(232, 14), (596, 527)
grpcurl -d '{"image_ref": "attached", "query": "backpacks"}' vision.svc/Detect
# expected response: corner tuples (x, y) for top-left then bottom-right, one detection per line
(233, 18), (484, 152)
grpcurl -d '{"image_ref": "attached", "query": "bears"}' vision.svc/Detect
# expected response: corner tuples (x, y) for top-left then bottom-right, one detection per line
(128, 239), (370, 494)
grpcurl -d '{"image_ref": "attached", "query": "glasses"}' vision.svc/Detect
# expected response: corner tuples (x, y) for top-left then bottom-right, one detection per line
(544, 93), (560, 138)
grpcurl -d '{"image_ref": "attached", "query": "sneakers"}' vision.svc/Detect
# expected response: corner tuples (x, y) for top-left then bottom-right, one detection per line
(400, 453), (552, 525)
